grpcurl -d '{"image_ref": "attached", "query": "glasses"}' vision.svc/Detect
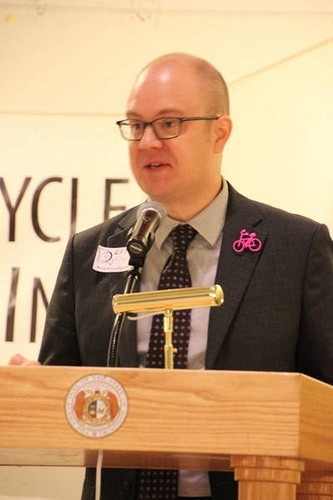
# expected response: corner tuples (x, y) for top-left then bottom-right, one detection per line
(116, 115), (219, 141)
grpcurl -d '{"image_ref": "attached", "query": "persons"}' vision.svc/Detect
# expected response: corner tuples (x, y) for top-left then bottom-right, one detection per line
(7, 49), (333, 500)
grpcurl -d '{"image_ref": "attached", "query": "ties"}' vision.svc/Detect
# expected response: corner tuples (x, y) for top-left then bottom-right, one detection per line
(136, 224), (197, 500)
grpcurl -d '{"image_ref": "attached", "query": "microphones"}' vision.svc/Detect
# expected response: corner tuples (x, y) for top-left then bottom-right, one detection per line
(125, 199), (167, 257)
